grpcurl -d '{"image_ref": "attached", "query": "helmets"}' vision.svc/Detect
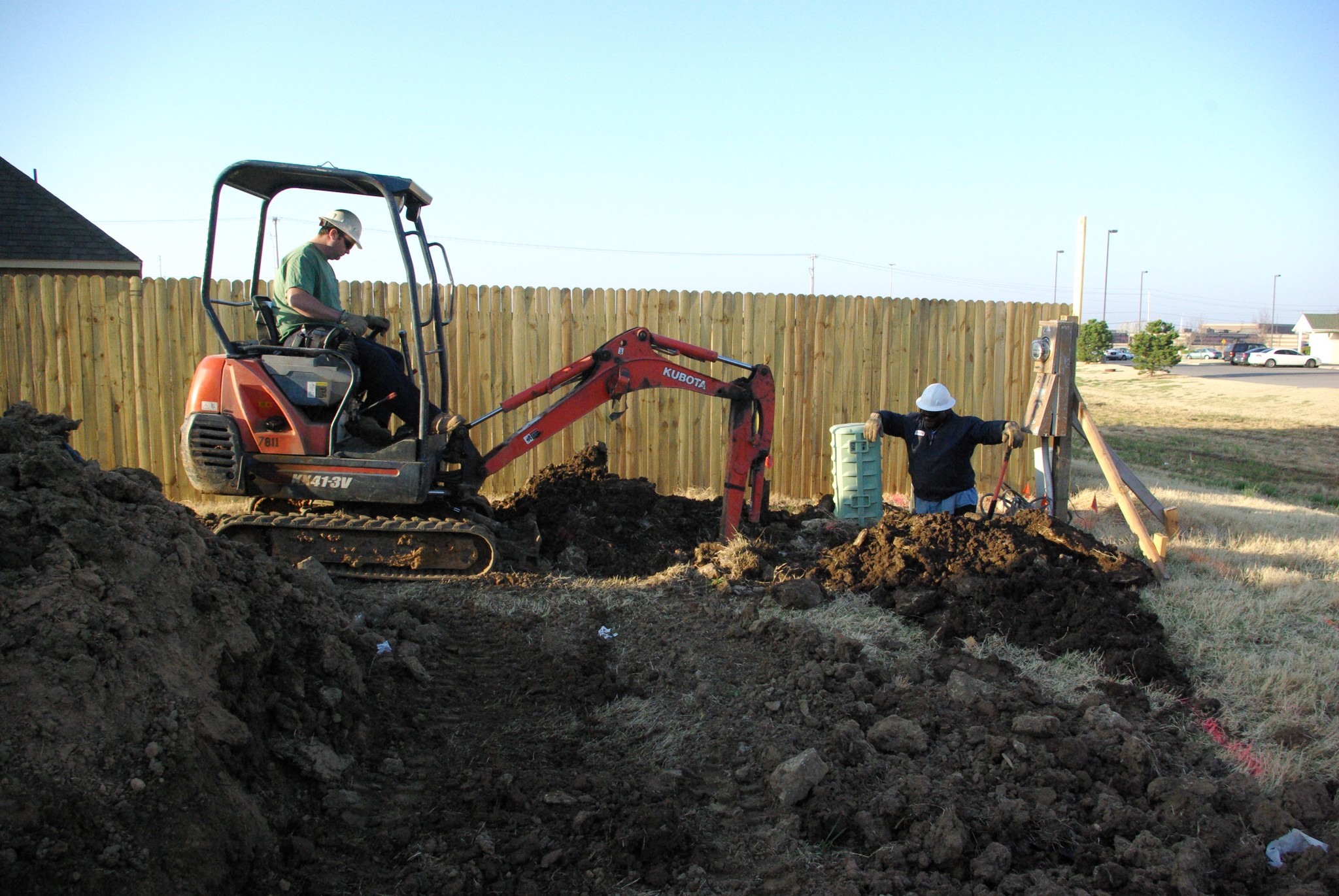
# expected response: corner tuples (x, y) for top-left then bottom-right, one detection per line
(916, 382), (956, 411)
(318, 209), (363, 250)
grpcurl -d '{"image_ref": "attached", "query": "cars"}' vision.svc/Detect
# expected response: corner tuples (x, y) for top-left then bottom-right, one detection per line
(1233, 347), (1321, 368)
(1105, 348), (1134, 361)
(1186, 348), (1223, 360)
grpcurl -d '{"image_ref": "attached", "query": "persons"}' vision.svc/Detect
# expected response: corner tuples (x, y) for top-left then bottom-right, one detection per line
(268, 209), (466, 448)
(863, 383), (1025, 517)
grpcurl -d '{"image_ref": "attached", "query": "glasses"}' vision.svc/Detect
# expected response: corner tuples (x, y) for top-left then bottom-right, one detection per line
(337, 232), (354, 251)
(920, 412), (940, 420)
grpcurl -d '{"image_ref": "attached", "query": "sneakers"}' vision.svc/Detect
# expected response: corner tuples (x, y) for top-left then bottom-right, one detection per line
(428, 411), (467, 433)
(359, 418), (391, 442)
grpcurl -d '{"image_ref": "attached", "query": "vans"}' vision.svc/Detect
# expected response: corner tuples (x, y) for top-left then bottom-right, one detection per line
(1224, 342), (1267, 366)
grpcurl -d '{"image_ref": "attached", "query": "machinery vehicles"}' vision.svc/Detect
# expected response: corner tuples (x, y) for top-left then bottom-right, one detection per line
(174, 158), (777, 582)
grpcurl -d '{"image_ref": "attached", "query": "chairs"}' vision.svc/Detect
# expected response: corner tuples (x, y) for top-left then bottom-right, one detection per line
(252, 296), (284, 347)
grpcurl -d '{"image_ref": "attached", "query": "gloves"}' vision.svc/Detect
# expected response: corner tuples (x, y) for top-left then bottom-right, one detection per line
(338, 310), (368, 337)
(365, 314), (390, 336)
(1001, 422), (1025, 449)
(863, 413), (885, 442)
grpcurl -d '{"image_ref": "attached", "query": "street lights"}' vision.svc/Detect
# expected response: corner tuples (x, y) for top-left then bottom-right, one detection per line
(1270, 274), (1281, 348)
(1138, 270), (1148, 333)
(1054, 249), (1065, 303)
(1103, 229), (1119, 322)
